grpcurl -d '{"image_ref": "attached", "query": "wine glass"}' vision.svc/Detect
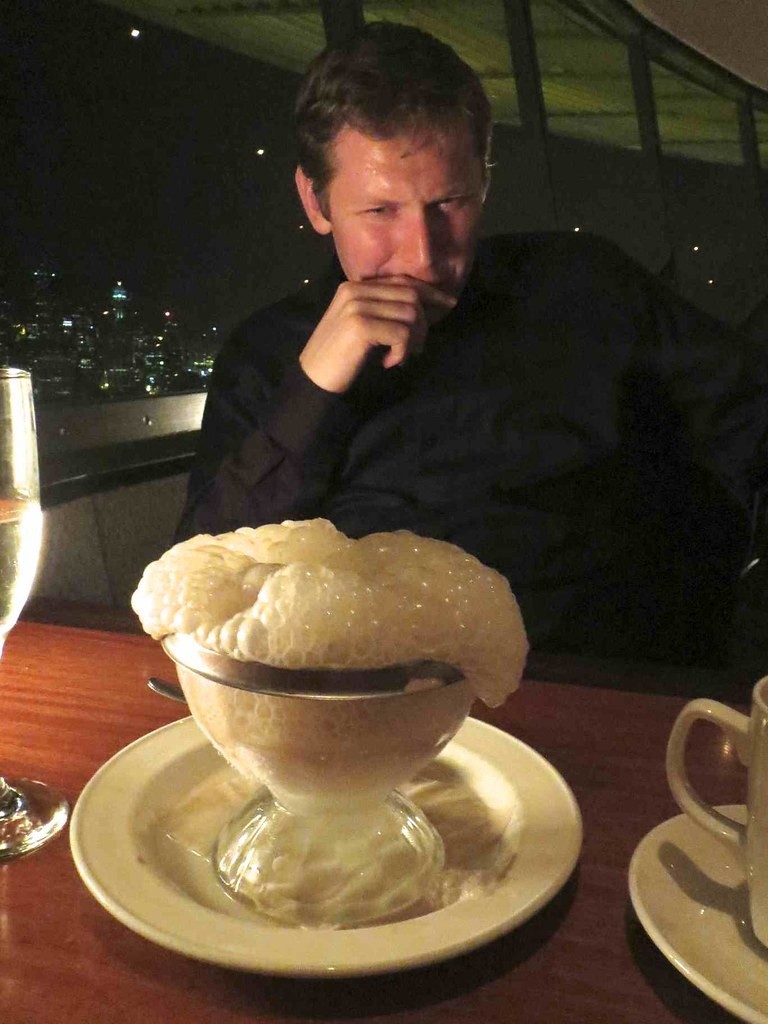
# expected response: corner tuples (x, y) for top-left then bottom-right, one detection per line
(159, 634), (476, 926)
(1, 367), (70, 861)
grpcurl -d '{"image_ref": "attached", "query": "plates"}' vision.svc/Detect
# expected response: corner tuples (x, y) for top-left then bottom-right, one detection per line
(66, 714), (583, 980)
(628, 804), (768, 1024)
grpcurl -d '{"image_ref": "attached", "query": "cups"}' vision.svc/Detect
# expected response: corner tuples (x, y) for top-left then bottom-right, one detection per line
(665, 676), (768, 948)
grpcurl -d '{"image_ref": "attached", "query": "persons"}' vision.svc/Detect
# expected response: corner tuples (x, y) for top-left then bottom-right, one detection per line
(175, 19), (767, 664)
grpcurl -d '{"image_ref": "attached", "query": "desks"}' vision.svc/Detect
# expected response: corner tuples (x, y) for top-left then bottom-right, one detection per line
(1, 593), (749, 1024)
